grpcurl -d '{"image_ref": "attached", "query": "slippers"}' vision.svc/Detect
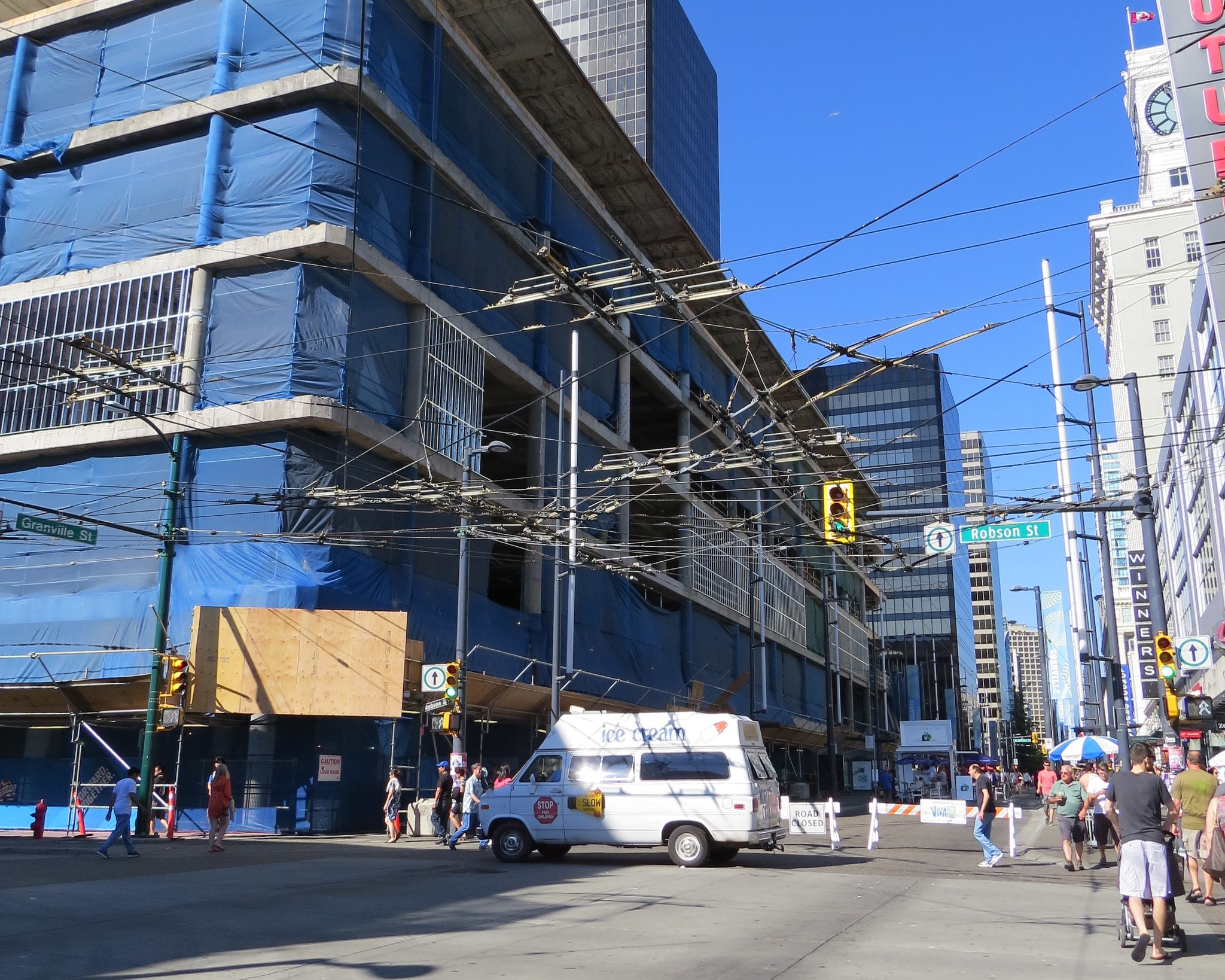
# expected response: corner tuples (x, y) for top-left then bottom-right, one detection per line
(1131, 933), (1150, 962)
(1150, 951), (1172, 959)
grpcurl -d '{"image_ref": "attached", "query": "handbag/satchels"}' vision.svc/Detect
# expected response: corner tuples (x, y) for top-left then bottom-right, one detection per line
(229, 801), (236, 822)
(1172, 837), (1184, 881)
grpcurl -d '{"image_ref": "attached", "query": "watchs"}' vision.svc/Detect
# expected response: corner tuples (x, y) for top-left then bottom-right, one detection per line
(1203, 794), (1225, 892)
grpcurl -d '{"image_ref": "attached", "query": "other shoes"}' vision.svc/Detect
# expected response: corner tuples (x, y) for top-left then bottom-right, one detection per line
(127, 851), (140, 857)
(989, 853), (1004, 867)
(479, 845), (491, 850)
(1049, 821), (1054, 826)
(1044, 817), (1049, 825)
(449, 845), (457, 850)
(1064, 863), (1074, 871)
(395, 832), (402, 841)
(1076, 860), (1084, 870)
(976, 860), (995, 867)
(435, 837), (447, 846)
(1100, 857), (1106, 865)
(384, 838), (396, 843)
(97, 850), (111, 859)
(213, 845), (224, 852)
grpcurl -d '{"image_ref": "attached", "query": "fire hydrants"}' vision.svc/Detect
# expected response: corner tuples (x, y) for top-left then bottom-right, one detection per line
(30, 798), (48, 838)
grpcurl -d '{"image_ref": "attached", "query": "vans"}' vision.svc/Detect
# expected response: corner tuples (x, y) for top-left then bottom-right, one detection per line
(476, 705), (789, 863)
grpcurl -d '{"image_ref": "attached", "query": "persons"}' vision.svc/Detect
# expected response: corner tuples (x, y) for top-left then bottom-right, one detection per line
(879, 760), (1165, 826)
(1170, 749), (1219, 905)
(1047, 764), (1090, 871)
(1207, 766), (1220, 779)
(968, 764), (1005, 867)
(1086, 763), (1120, 865)
(448, 762), (492, 851)
(207, 764), (234, 852)
(205, 755), (230, 836)
(431, 760), (453, 845)
(493, 763), (511, 788)
(95, 765), (151, 859)
(546, 768), (561, 782)
(383, 768), (402, 843)
(1102, 740), (1180, 960)
(149, 764), (169, 836)
(449, 767), (467, 840)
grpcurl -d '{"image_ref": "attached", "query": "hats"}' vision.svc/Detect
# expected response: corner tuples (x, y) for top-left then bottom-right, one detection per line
(436, 761), (449, 767)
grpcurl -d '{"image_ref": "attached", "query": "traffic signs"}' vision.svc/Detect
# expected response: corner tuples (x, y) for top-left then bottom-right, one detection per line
(958, 520), (1051, 544)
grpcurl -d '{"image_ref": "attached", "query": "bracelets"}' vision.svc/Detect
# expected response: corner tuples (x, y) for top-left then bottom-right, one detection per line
(433, 801), (437, 804)
(1172, 822), (1177, 825)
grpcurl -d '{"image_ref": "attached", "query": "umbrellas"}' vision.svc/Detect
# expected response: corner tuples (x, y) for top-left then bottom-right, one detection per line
(893, 754), (950, 766)
(1047, 734), (1120, 763)
(961, 752), (1000, 767)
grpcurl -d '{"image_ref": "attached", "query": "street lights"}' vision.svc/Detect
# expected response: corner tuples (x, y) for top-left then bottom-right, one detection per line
(1072, 372), (1179, 751)
(451, 439), (515, 803)
(100, 401), (181, 837)
(1010, 585), (1055, 772)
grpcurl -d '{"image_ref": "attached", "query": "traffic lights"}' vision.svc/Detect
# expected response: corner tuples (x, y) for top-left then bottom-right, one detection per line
(444, 662), (460, 699)
(169, 655), (190, 698)
(823, 479), (856, 547)
(1182, 695), (1213, 720)
(1154, 631), (1178, 680)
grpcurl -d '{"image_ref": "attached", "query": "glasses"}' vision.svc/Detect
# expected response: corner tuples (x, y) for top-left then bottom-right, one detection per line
(1061, 772), (1067, 774)
(1213, 772), (1217, 775)
(1043, 764), (1050, 766)
(438, 766), (442, 768)
(1143, 755), (1156, 761)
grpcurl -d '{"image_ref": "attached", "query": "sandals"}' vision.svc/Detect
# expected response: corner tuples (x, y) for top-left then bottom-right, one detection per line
(1204, 896), (1217, 905)
(1186, 888), (1202, 900)
